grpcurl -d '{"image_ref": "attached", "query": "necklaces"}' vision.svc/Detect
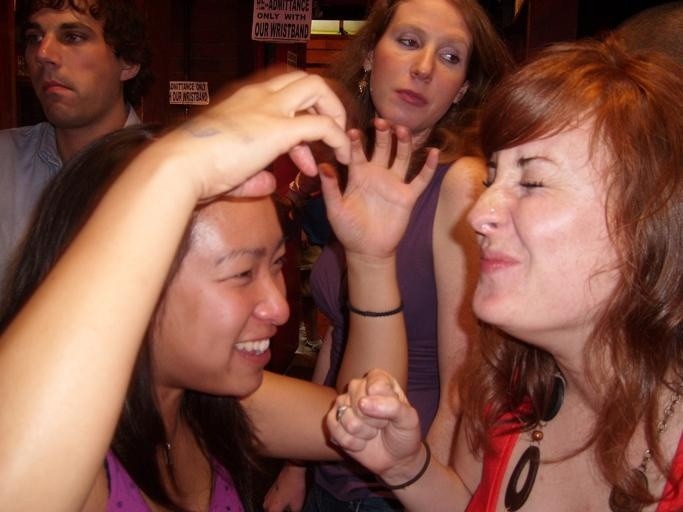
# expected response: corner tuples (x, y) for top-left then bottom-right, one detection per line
(504, 393), (682, 512)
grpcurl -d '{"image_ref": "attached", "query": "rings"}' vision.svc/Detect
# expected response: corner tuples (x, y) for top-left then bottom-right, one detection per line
(336, 405), (350, 420)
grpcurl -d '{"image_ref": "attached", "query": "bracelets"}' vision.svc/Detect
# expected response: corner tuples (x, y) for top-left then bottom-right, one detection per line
(378, 440), (431, 488)
(347, 302), (404, 317)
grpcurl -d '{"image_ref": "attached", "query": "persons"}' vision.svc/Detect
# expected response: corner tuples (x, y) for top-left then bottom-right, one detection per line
(0, 69), (440, 512)
(327, 39), (682, 512)
(259, 1), (518, 512)
(0, 2), (154, 263)
